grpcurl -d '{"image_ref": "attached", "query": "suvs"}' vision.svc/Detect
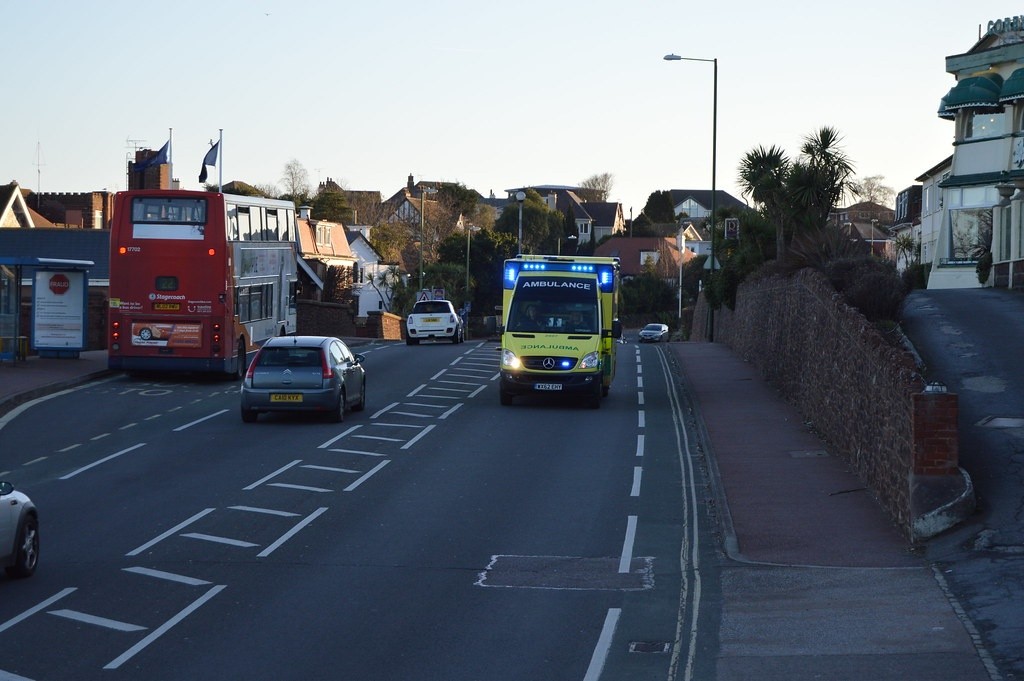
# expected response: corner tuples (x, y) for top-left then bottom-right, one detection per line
(406, 300), (465, 344)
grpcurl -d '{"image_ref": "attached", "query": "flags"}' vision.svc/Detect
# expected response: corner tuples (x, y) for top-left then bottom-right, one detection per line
(198, 140), (219, 183)
(132, 140), (169, 172)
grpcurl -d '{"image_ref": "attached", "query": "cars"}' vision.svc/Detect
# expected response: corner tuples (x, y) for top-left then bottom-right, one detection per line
(0, 481), (41, 584)
(240, 333), (366, 424)
(638, 323), (670, 343)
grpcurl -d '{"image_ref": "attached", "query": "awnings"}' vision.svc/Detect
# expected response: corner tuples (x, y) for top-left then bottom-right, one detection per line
(999, 68), (1024, 103)
(936, 76), (1002, 121)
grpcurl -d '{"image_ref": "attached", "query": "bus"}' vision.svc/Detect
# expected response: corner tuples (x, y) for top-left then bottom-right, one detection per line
(108, 189), (298, 380)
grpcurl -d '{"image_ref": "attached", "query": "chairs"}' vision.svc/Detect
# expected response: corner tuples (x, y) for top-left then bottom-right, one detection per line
(523, 305), (540, 322)
(307, 353), (320, 364)
(278, 348), (290, 360)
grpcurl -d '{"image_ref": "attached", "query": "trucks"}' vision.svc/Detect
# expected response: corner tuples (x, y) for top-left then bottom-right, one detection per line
(499, 253), (622, 414)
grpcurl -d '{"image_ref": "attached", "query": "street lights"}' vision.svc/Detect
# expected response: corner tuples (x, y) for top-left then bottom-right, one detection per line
(466, 226), (482, 342)
(664, 53), (719, 342)
(418, 188), (439, 300)
